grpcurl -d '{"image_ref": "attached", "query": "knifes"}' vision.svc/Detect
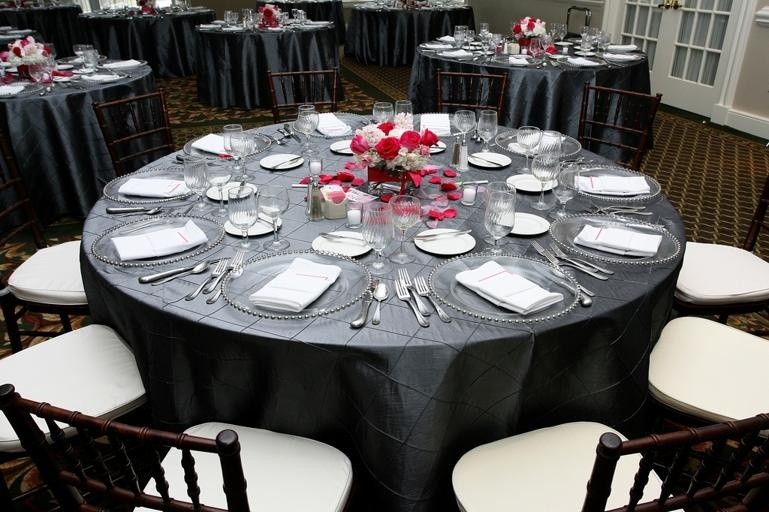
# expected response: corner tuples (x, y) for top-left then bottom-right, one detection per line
(347, 280), (380, 327)
(529, 262), (593, 307)
(416, 229), (472, 240)
(469, 154), (503, 167)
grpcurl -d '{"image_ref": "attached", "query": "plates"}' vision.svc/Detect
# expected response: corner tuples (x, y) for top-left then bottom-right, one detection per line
(330, 139), (361, 156)
(222, 252), (373, 322)
(206, 180), (259, 200)
(468, 152), (512, 169)
(96, 60), (148, 71)
(428, 141), (447, 155)
(413, 229), (476, 256)
(0, 84), (43, 97)
(507, 174), (559, 192)
(495, 213), (550, 236)
(92, 212), (223, 268)
(416, 112), (476, 138)
(429, 253), (581, 324)
(182, 131), (270, 161)
(259, 152), (304, 174)
(85, 71), (126, 84)
(223, 210), (286, 237)
(296, 111), (371, 140)
(104, 168), (202, 202)
(310, 230), (373, 260)
(549, 213), (681, 264)
(558, 163), (662, 201)
(495, 129), (581, 158)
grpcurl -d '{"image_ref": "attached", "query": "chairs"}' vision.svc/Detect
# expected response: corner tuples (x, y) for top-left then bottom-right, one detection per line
(0, 322), (145, 508)
(0, 383), (354, 511)
(674, 174), (768, 324)
(0, 240), (91, 352)
(448, 415), (769, 512)
(647, 313), (768, 444)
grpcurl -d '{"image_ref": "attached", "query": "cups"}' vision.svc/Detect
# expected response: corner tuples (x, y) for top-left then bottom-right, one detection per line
(15, 0), (21, 10)
(101, 0), (191, 19)
(484, 182), (514, 209)
(371, 100), (413, 124)
(460, 182), (479, 206)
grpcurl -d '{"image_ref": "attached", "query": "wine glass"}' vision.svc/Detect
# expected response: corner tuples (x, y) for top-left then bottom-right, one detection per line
(454, 110), (476, 145)
(516, 125), (579, 219)
(452, 22), (641, 68)
(27, 43), (107, 93)
(297, 102), (322, 159)
(477, 110), (498, 153)
(263, 184), (292, 251)
(182, 159), (214, 213)
(363, 201), (393, 276)
(230, 129), (260, 184)
(219, 8), (309, 31)
(222, 124), (244, 181)
(225, 184), (260, 253)
(387, 194), (421, 264)
(481, 181), (516, 257)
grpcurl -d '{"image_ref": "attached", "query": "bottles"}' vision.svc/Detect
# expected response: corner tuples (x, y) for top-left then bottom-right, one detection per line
(447, 140), (469, 172)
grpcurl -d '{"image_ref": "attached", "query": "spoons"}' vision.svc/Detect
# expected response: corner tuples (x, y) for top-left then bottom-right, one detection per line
(152, 260), (210, 290)
(549, 210), (662, 231)
(104, 203), (164, 219)
(270, 122), (298, 146)
(371, 283), (390, 325)
(206, 264), (245, 306)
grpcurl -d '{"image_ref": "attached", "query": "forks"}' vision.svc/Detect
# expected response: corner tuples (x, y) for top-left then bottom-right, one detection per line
(531, 239), (614, 296)
(138, 255), (209, 285)
(393, 279), (431, 328)
(397, 267), (432, 318)
(202, 251), (244, 294)
(186, 257), (228, 299)
(579, 200), (654, 217)
(412, 275), (453, 324)
